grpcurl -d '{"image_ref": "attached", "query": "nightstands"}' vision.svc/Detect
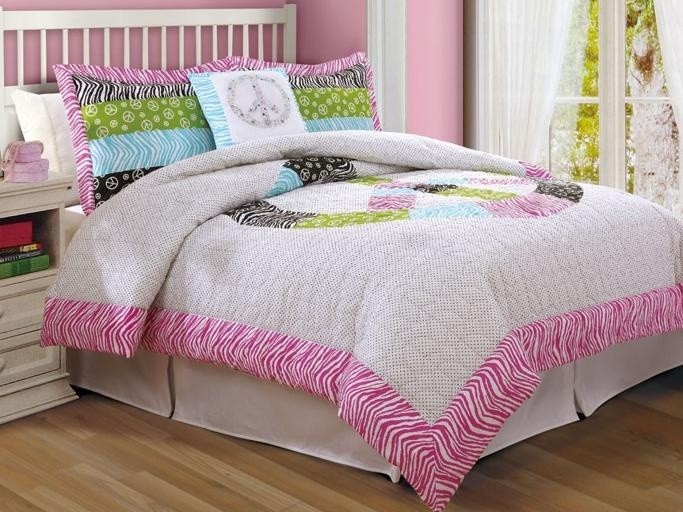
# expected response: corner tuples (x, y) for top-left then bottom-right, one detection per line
(0, 168), (82, 427)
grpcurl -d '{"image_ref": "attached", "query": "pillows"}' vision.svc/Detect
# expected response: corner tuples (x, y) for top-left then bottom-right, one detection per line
(51, 57), (381, 213)
(12, 87), (76, 178)
(187, 68), (313, 153)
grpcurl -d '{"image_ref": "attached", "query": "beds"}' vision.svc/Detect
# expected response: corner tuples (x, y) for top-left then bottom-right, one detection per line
(0, 0), (683, 512)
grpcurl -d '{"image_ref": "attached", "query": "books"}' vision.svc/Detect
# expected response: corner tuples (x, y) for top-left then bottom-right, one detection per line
(0, 249), (43, 265)
(0, 253), (50, 280)
(0, 219), (32, 249)
(0, 240), (42, 257)
